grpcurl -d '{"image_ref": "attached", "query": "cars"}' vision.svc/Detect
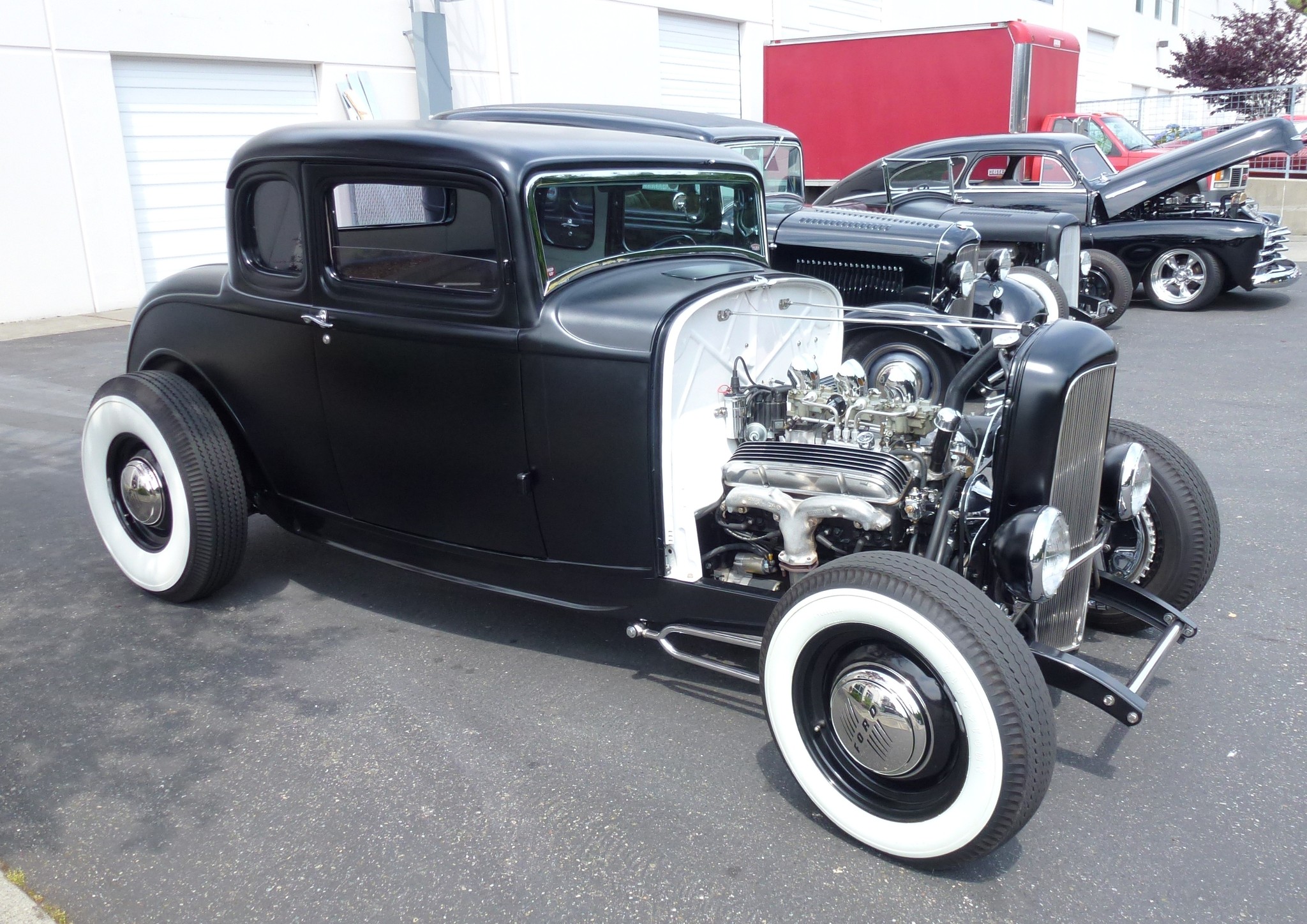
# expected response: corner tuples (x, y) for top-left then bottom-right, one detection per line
(76, 103), (1219, 874)
(1159, 116), (1307, 179)
(810, 116), (1303, 312)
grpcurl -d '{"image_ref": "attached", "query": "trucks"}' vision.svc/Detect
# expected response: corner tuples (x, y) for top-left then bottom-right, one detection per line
(759, 20), (1215, 194)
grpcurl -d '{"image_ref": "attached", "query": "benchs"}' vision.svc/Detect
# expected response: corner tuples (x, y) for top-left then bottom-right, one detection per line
(341, 247), (503, 289)
